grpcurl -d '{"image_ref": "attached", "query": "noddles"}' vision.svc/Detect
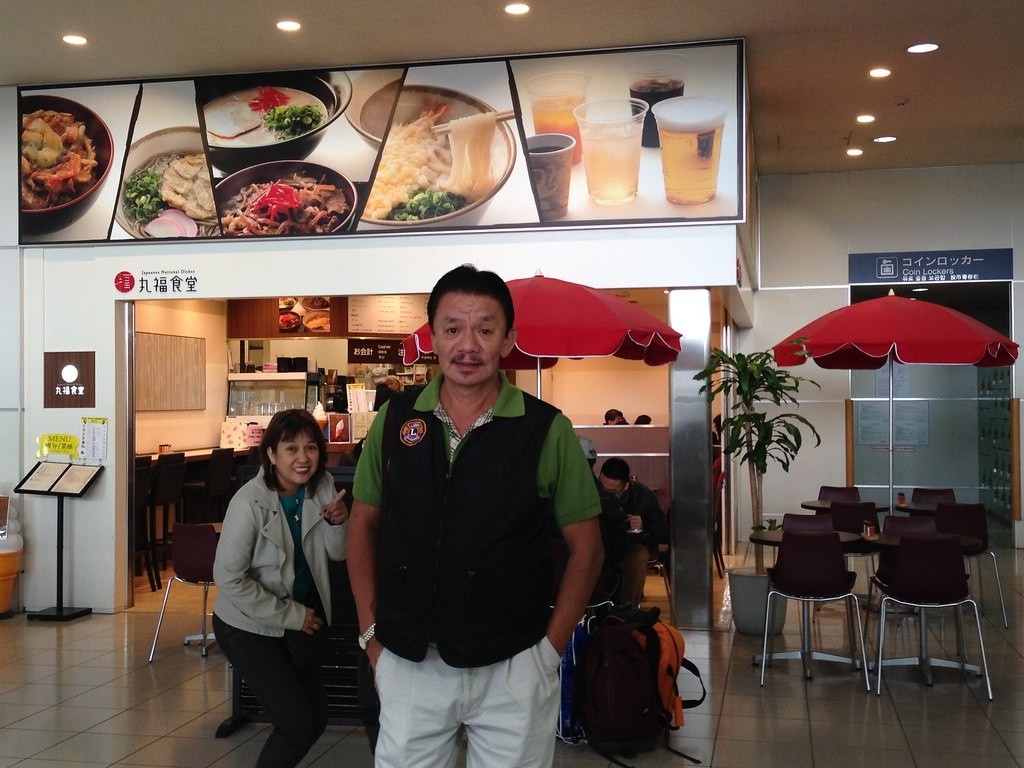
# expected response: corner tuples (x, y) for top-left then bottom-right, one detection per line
(123, 150), (220, 237)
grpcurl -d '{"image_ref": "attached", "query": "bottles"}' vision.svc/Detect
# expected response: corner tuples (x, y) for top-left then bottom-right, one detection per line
(159, 444), (170, 454)
(864, 520), (875, 537)
(898, 492), (905, 504)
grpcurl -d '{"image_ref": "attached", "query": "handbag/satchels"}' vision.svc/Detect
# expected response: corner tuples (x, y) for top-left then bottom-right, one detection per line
(557, 614), (587, 745)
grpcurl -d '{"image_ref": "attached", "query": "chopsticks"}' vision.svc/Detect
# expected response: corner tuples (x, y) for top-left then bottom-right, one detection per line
(430, 110), (515, 137)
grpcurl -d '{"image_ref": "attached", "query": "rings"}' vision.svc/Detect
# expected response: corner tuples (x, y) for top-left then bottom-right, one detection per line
(313, 624), (316, 629)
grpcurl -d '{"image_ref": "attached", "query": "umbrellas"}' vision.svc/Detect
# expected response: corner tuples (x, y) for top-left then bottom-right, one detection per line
(771, 289), (1020, 516)
(400, 269), (685, 401)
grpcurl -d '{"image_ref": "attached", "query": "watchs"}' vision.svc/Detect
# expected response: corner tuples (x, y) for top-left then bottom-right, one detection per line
(358, 623), (376, 650)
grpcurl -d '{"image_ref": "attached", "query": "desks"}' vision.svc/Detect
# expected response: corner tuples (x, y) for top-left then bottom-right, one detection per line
(801, 500), (890, 613)
(749, 530), (861, 670)
(888, 504), (964, 614)
(135, 448), (249, 466)
(863, 532), (983, 676)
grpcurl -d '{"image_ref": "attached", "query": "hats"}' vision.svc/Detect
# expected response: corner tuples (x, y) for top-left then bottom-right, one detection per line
(577, 435), (598, 460)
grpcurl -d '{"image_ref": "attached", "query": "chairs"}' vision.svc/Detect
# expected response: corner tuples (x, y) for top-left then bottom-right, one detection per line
(712, 469), (726, 579)
(760, 486), (1008, 702)
(643, 489), (675, 626)
(149, 523), (218, 663)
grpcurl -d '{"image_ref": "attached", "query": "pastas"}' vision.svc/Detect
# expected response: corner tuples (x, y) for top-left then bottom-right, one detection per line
(420, 110), (494, 202)
(221, 174), (336, 235)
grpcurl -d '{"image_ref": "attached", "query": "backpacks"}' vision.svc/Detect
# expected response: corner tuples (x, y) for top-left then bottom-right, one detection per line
(569, 599), (707, 767)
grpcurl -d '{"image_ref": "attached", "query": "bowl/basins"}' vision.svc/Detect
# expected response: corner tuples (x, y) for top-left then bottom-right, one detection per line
(115, 127), (200, 238)
(200, 71), (353, 178)
(214, 161), (358, 235)
(303, 310), (330, 332)
(360, 85), (517, 226)
(21, 95), (114, 234)
(345, 68), (404, 152)
(279, 311), (301, 332)
(279, 298), (298, 312)
(299, 297), (330, 312)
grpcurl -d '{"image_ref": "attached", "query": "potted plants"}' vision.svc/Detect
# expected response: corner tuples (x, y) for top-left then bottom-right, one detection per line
(694, 337), (822, 635)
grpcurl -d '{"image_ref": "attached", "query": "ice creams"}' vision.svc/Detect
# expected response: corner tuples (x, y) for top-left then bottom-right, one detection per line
(336, 420), (344, 437)
(311, 401), (327, 433)
(0, 507), (24, 612)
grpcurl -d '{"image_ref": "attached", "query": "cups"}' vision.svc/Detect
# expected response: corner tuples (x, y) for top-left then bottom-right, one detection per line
(526, 133), (576, 221)
(621, 51), (693, 148)
(572, 97), (649, 207)
(525, 72), (592, 165)
(232, 383), (256, 401)
(237, 402), (309, 416)
(651, 95), (732, 205)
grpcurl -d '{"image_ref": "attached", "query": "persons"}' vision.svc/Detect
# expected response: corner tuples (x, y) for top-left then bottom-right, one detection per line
(712, 414), (721, 459)
(371, 367), (401, 411)
(212, 409), (350, 768)
(577, 435), (666, 608)
(338, 436), (366, 467)
(604, 409), (652, 425)
(346, 266), (604, 768)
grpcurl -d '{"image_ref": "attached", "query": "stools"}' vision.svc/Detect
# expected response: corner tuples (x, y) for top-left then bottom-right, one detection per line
(133, 446), (261, 592)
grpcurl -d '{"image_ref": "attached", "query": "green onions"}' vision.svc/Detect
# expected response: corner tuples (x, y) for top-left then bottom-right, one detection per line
(123, 172), (162, 220)
(261, 103), (320, 141)
(387, 189), (468, 220)
